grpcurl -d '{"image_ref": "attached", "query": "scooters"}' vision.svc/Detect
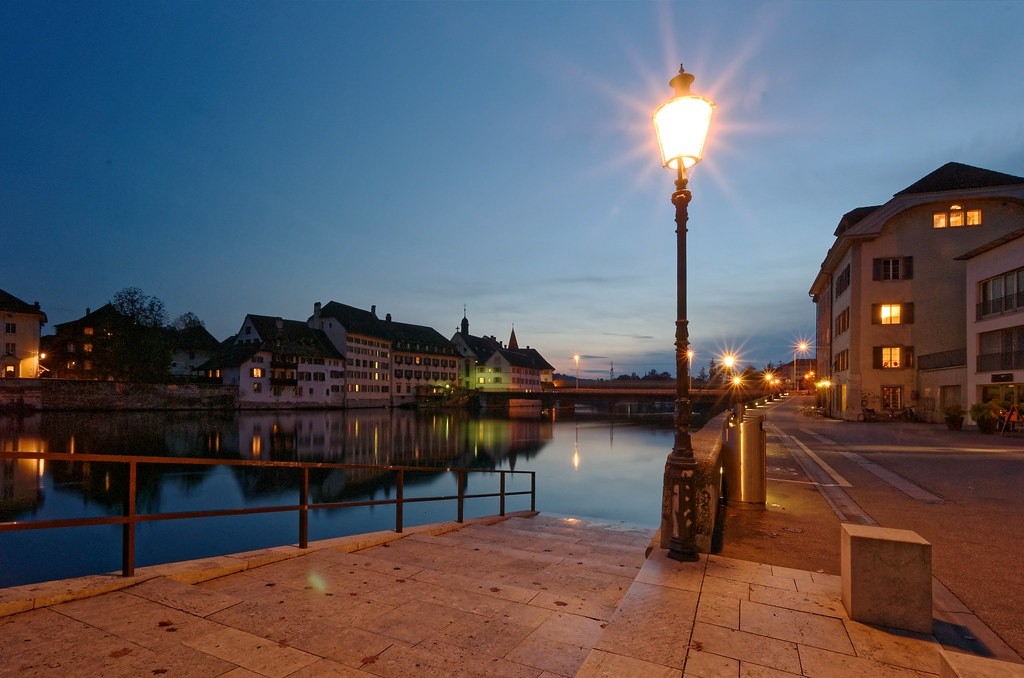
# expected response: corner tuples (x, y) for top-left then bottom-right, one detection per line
(886, 405), (917, 423)
(857, 404), (878, 424)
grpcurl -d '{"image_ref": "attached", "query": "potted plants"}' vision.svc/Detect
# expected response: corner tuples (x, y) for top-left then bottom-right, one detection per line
(944, 402), (965, 430)
(969, 400), (1002, 434)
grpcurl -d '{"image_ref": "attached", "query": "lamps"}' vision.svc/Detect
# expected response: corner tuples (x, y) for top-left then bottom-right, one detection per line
(39, 353), (45, 360)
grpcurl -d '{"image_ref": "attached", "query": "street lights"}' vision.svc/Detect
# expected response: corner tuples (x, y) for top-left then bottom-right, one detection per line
(687, 351), (694, 390)
(793, 342), (809, 390)
(575, 354), (580, 388)
(649, 60), (717, 564)
(825, 381), (833, 419)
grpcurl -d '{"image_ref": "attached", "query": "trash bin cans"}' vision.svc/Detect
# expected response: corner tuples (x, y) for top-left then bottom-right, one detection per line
(722, 403), (766, 502)
(771, 393), (773, 401)
